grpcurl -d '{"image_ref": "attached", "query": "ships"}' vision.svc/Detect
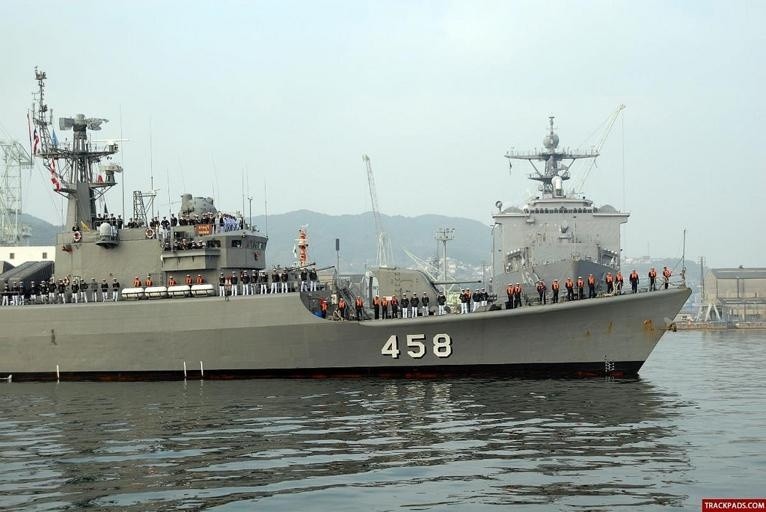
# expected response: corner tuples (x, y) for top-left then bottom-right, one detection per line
(0, 65), (693, 381)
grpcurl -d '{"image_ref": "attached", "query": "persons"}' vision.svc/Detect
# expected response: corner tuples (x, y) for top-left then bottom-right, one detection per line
(322, 267), (672, 321)
(92, 208), (245, 252)
(1, 264), (319, 306)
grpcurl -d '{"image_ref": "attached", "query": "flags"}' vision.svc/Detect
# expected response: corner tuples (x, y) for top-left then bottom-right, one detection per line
(49, 157), (61, 191)
(32, 126), (40, 156)
